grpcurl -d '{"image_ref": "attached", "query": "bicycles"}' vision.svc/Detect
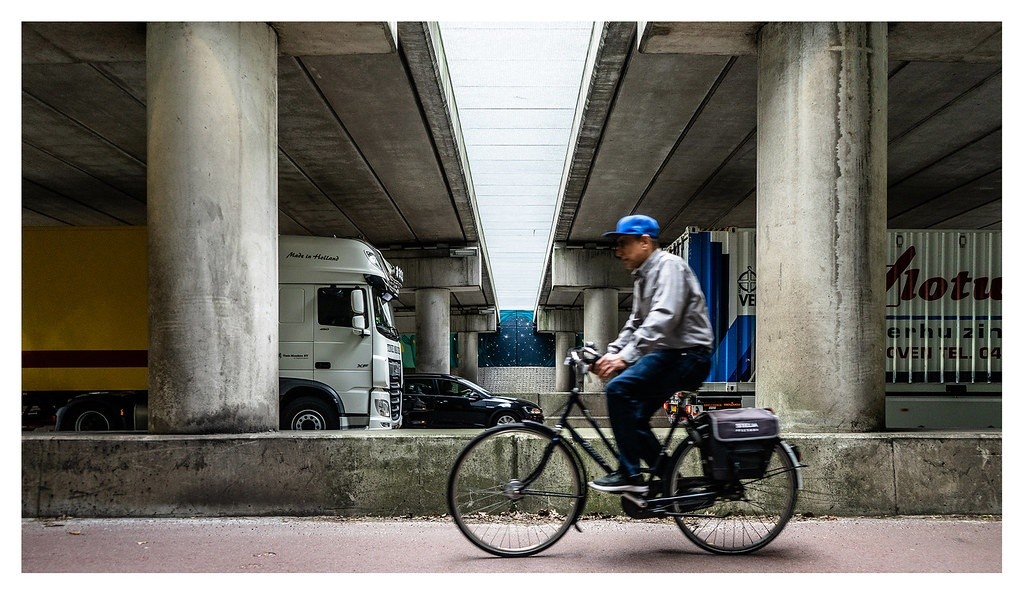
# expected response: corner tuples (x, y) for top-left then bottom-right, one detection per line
(446, 341), (812, 558)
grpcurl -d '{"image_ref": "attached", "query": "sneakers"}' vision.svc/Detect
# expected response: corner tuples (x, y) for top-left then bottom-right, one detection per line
(586, 469), (650, 492)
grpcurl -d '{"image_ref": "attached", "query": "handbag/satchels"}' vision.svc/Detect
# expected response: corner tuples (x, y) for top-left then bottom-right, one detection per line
(695, 408), (779, 479)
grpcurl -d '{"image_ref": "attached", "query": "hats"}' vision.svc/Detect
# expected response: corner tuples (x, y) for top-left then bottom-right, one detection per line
(600, 215), (660, 238)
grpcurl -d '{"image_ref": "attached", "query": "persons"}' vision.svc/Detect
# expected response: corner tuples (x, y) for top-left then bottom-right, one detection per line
(584, 215), (716, 494)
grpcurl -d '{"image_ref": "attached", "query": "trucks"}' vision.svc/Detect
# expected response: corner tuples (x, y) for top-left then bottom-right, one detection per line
(662, 225), (1002, 433)
(22, 223), (414, 433)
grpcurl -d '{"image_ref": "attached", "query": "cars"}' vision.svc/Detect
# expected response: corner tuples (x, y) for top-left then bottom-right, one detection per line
(400, 371), (545, 430)
